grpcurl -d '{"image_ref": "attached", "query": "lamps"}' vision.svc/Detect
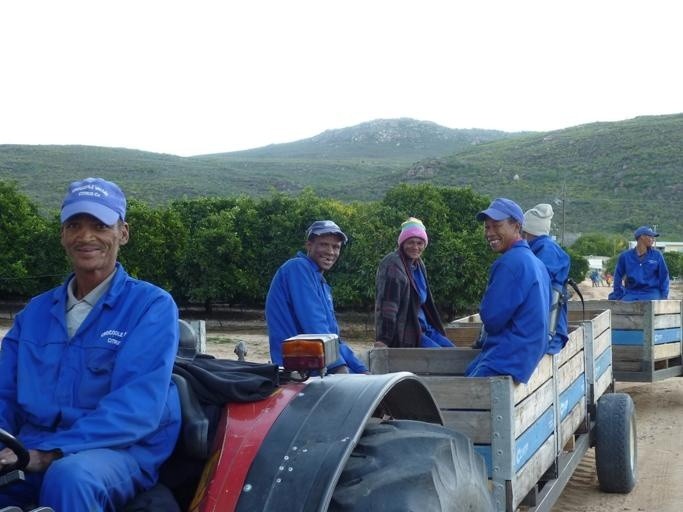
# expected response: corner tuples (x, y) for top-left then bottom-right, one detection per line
(162, 318), (210, 512)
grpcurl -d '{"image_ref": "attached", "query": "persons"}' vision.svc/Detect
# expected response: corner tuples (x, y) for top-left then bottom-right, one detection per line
(589, 270), (598, 288)
(594, 269), (605, 287)
(264, 219), (372, 375)
(1, 177), (182, 511)
(471, 202), (570, 356)
(374, 215), (458, 348)
(608, 226), (669, 303)
(605, 271), (612, 287)
(463, 197), (552, 385)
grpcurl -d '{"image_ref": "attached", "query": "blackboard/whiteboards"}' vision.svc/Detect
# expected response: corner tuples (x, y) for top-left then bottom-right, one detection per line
(0, 320), (498, 512)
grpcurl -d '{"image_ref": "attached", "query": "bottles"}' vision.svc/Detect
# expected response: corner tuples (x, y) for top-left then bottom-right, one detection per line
(398, 217), (428, 248)
(475, 198), (554, 237)
(60, 178), (127, 227)
(635, 226), (659, 238)
(306, 221), (348, 246)
